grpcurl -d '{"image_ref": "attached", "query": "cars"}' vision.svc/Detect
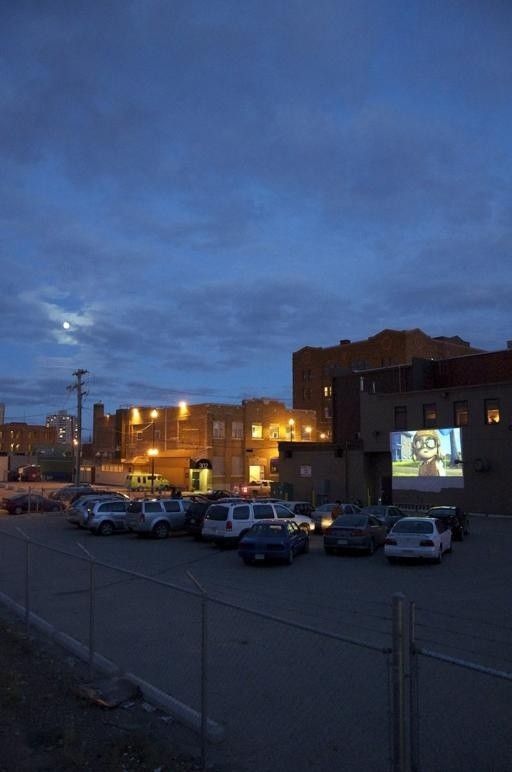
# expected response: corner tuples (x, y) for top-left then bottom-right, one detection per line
(322, 512), (390, 557)
(11, 465), (42, 482)
(238, 519), (310, 567)
(425, 505), (470, 542)
(1, 493), (65, 515)
(46, 482), (108, 502)
(384, 517), (454, 566)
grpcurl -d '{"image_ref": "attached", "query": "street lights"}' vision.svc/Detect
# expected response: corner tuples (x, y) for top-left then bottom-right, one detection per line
(147, 409), (159, 494)
(289, 418), (295, 441)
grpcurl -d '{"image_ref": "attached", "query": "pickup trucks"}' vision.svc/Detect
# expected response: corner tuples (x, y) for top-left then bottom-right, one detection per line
(232, 479), (275, 497)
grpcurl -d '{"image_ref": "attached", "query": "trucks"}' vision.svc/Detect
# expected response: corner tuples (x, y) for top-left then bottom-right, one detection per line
(124, 473), (170, 492)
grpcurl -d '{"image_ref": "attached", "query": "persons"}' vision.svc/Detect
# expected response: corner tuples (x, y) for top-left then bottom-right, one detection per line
(331, 500), (344, 520)
(411, 429), (446, 478)
(154, 488), (181, 501)
